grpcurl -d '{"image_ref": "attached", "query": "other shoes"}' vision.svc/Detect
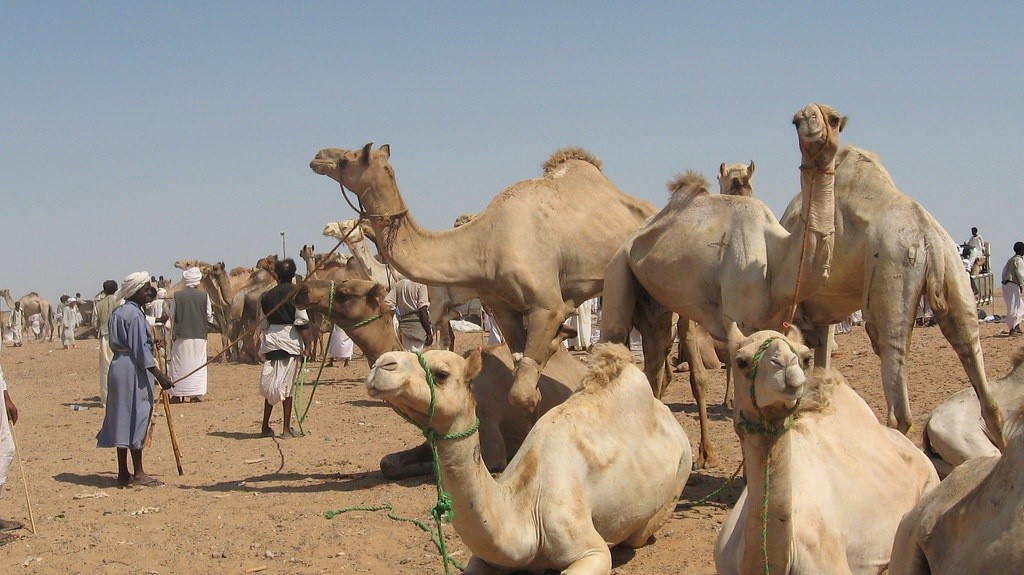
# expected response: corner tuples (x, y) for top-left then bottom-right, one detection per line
(1010, 329), (1023, 337)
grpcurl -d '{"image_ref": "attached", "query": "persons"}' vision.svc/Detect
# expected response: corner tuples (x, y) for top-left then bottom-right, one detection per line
(91, 280), (125, 406)
(562, 296), (602, 353)
(57, 293), (83, 349)
(259, 259), (311, 439)
(170, 267), (212, 404)
(97, 272), (174, 488)
(384, 279), (433, 354)
(0, 365), (25, 543)
(141, 274), (171, 384)
(955, 227), (985, 285)
(916, 295), (936, 327)
(835, 310), (863, 334)
(324, 324), (353, 367)
(7, 302), (25, 347)
(1001, 242), (1024, 337)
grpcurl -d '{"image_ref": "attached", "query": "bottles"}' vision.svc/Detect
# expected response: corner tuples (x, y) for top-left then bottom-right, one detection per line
(70, 405), (89, 411)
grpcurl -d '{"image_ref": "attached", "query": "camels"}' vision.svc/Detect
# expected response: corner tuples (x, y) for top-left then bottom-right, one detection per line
(0, 288), (54, 343)
(153, 103), (1024, 575)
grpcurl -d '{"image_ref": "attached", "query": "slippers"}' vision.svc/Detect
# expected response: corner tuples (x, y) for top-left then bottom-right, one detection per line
(262, 427), (275, 436)
(118, 475), (134, 485)
(281, 430), (304, 439)
(134, 473), (161, 487)
(0, 519), (23, 531)
(0, 532), (17, 547)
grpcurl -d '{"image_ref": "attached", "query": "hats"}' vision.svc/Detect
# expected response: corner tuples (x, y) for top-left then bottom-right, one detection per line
(182, 266), (202, 287)
(67, 297), (76, 306)
(120, 270), (150, 300)
(158, 288), (167, 300)
